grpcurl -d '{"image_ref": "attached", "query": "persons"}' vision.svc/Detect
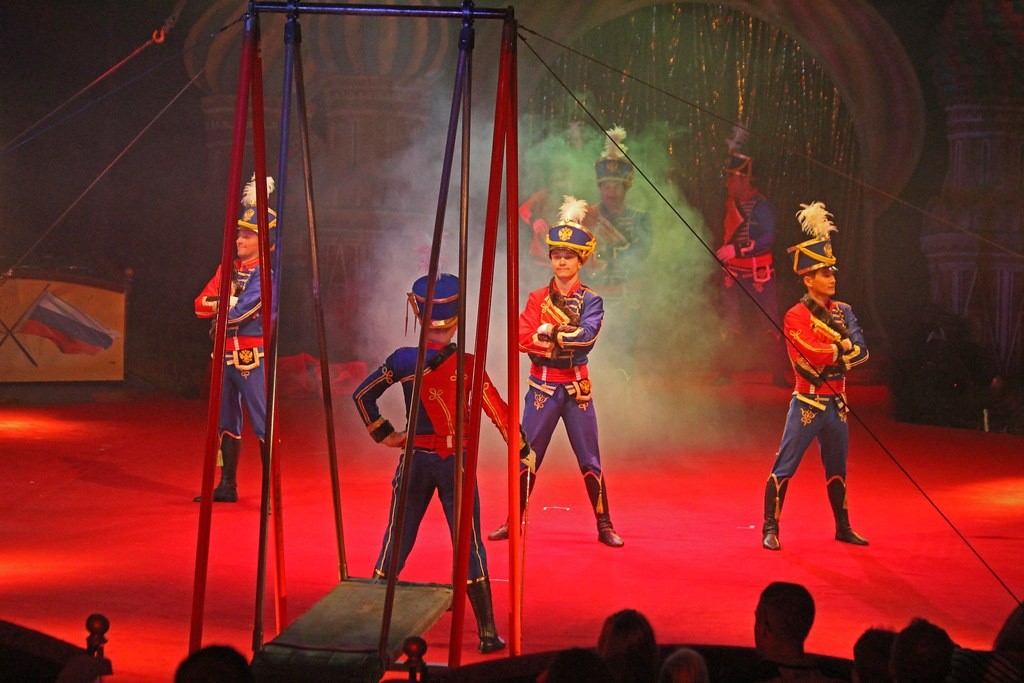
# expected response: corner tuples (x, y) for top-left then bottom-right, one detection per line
(706, 106), (796, 392)
(487, 194), (625, 548)
(538, 577), (1024, 683)
(175, 645), (255, 683)
(352, 277), (538, 654)
(520, 171), (574, 263)
(763, 201), (872, 550)
(571, 127), (652, 371)
(192, 172), (283, 507)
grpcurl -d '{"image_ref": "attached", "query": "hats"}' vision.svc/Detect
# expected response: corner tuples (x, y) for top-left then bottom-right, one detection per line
(546, 195), (597, 264)
(237, 171), (277, 240)
(594, 123), (635, 185)
(725, 121), (763, 181)
(405, 231), (460, 336)
(786, 201), (837, 274)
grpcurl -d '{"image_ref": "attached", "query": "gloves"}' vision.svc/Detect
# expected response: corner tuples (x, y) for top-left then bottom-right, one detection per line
(536, 323), (551, 335)
(842, 337), (853, 350)
(520, 447), (537, 474)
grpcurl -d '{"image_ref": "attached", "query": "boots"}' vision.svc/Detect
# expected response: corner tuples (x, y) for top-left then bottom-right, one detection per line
(259, 436), (282, 515)
(826, 475), (869, 545)
(466, 577), (506, 653)
(488, 469), (537, 541)
(762, 473), (789, 550)
(193, 430), (241, 502)
(764, 328), (792, 389)
(711, 328), (741, 386)
(583, 470), (624, 547)
